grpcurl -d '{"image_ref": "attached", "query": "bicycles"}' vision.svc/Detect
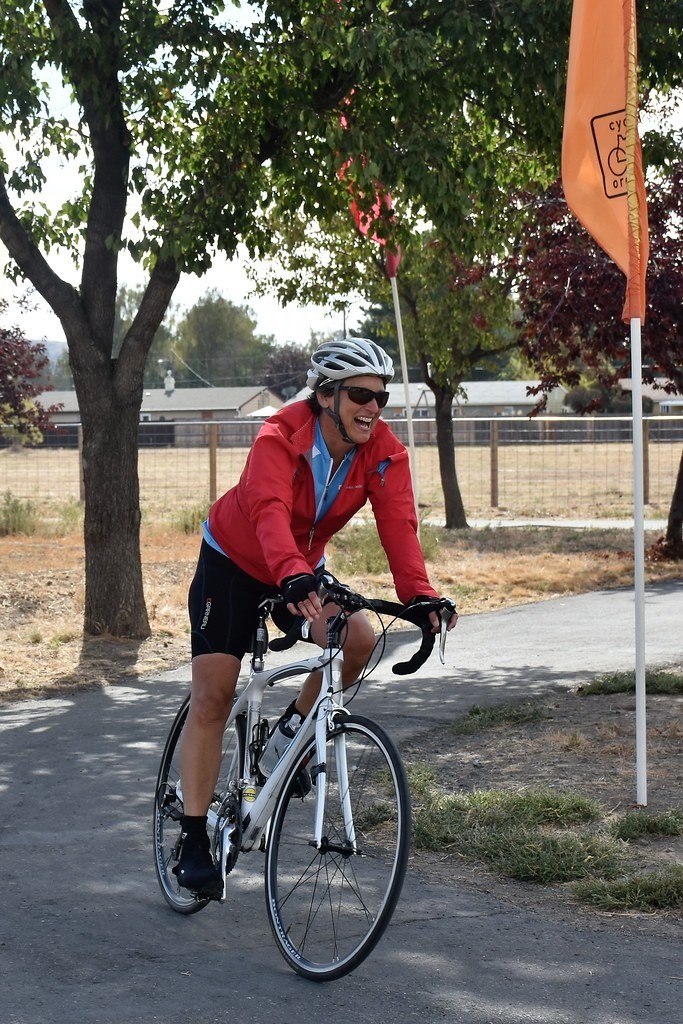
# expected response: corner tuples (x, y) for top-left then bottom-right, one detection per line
(150, 569), (458, 984)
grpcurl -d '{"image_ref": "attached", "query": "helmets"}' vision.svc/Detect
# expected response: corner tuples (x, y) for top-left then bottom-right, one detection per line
(305, 337), (395, 390)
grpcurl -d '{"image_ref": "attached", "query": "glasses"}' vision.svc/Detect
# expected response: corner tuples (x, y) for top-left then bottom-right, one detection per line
(331, 386), (389, 408)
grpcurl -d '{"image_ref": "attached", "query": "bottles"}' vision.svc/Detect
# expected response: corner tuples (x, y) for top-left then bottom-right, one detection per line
(258, 714), (302, 779)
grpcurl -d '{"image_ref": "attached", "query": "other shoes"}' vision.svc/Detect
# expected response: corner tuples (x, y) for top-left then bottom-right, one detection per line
(292, 770), (311, 799)
(179, 832), (224, 892)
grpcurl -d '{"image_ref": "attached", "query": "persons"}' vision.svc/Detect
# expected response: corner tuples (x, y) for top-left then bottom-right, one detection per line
(173, 337), (460, 896)
